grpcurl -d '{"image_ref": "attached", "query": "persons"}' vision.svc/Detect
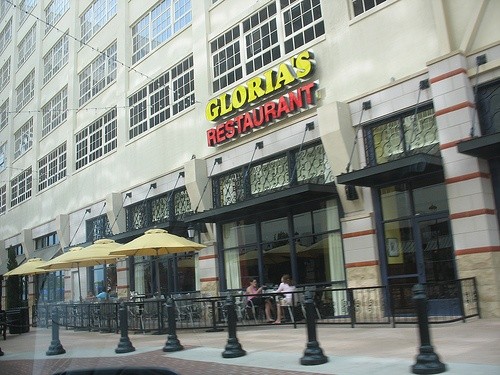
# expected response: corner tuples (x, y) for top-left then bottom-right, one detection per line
(86, 291), (98, 302)
(92, 286), (112, 301)
(246, 278), (276, 322)
(272, 273), (298, 324)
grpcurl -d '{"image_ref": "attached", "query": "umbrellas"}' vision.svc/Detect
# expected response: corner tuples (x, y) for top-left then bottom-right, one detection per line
(35, 246), (88, 332)
(3, 257), (62, 328)
(59, 238), (128, 332)
(108, 213), (215, 333)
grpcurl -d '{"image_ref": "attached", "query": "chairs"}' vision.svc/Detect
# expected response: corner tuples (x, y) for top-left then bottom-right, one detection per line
(0, 305), (28, 340)
(61, 285), (319, 331)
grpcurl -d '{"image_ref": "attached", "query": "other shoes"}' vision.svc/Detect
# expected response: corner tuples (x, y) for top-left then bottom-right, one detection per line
(266, 319), (275, 323)
(272, 321), (281, 324)
